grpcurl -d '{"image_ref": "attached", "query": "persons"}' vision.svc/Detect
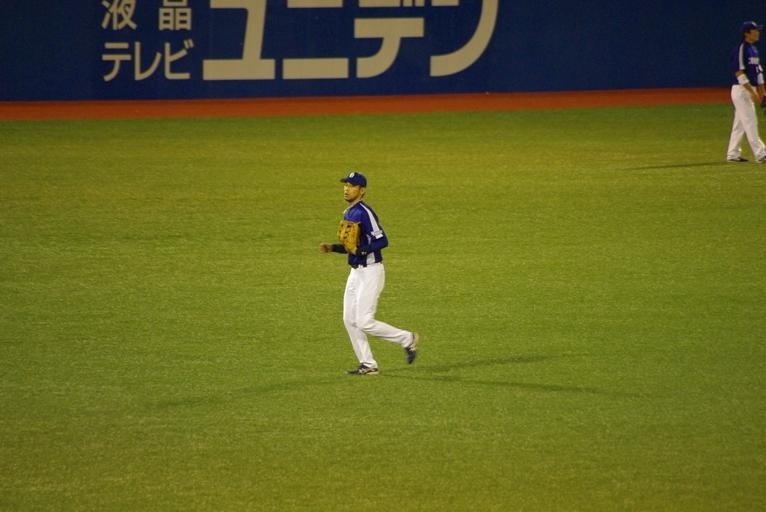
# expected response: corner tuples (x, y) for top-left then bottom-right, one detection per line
(725, 21), (766, 164)
(319, 171), (419, 376)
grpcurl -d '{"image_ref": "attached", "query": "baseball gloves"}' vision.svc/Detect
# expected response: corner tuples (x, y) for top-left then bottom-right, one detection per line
(336, 220), (360, 251)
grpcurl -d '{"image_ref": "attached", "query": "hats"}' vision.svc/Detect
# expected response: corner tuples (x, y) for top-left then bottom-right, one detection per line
(739, 21), (763, 32)
(340, 172), (367, 188)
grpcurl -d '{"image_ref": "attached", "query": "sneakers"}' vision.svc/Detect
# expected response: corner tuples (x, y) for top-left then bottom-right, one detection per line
(727, 157), (748, 162)
(347, 365), (380, 375)
(758, 156), (766, 162)
(405, 332), (419, 364)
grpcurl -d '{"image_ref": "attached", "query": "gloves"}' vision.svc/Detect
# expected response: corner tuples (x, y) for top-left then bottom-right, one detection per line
(318, 243), (332, 254)
